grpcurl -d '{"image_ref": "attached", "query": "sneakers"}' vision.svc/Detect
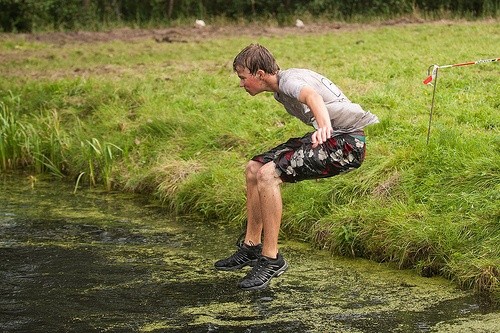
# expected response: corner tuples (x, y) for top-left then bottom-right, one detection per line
(238, 253), (288, 290)
(214, 242), (263, 269)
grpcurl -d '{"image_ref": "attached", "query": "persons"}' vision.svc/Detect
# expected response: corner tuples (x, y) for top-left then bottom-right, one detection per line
(212, 43), (380, 291)
